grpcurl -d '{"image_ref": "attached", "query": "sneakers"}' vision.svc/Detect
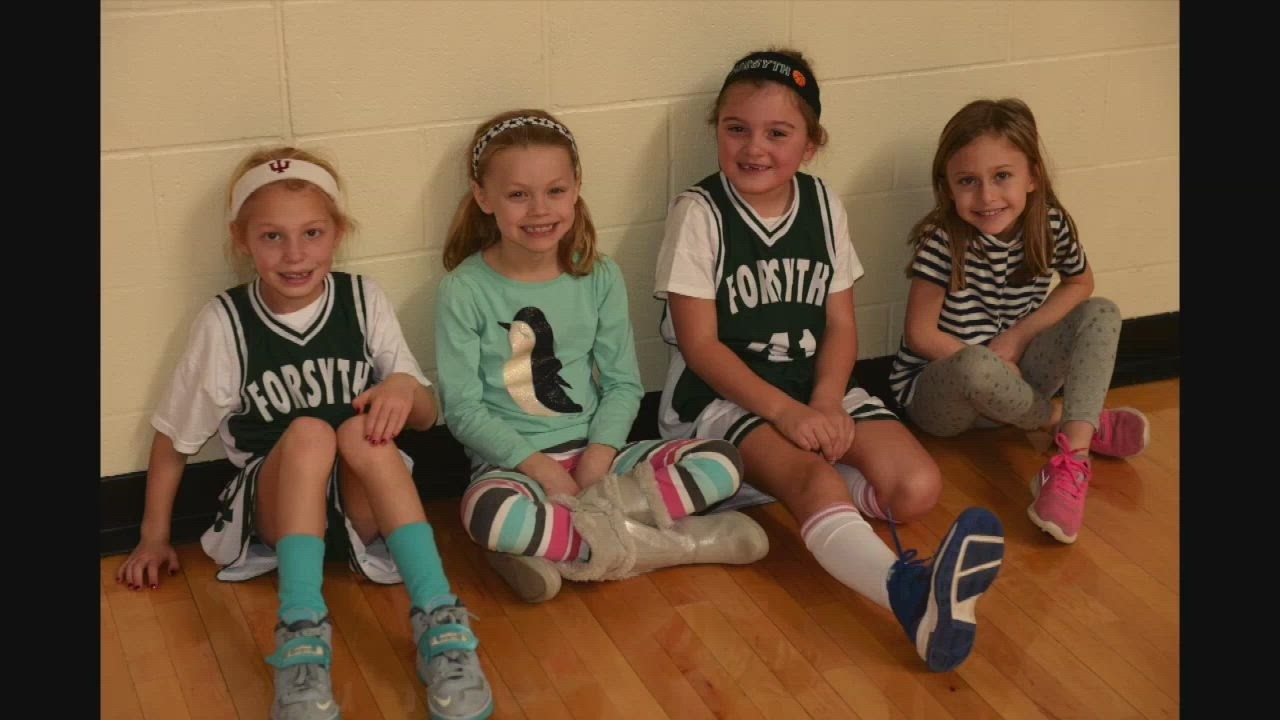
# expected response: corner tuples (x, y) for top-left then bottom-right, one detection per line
(1027, 431), (1093, 548)
(1089, 406), (1151, 460)
(271, 619), (342, 720)
(885, 499), (1007, 676)
(409, 597), (496, 720)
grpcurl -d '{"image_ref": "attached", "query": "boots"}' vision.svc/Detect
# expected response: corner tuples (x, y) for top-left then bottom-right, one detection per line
(546, 494), (772, 584)
(484, 459), (675, 607)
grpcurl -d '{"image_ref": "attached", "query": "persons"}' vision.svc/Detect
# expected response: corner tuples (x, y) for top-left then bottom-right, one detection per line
(118, 148), (496, 720)
(655, 49), (1006, 672)
(889, 96), (1150, 545)
(433, 109), (771, 601)
